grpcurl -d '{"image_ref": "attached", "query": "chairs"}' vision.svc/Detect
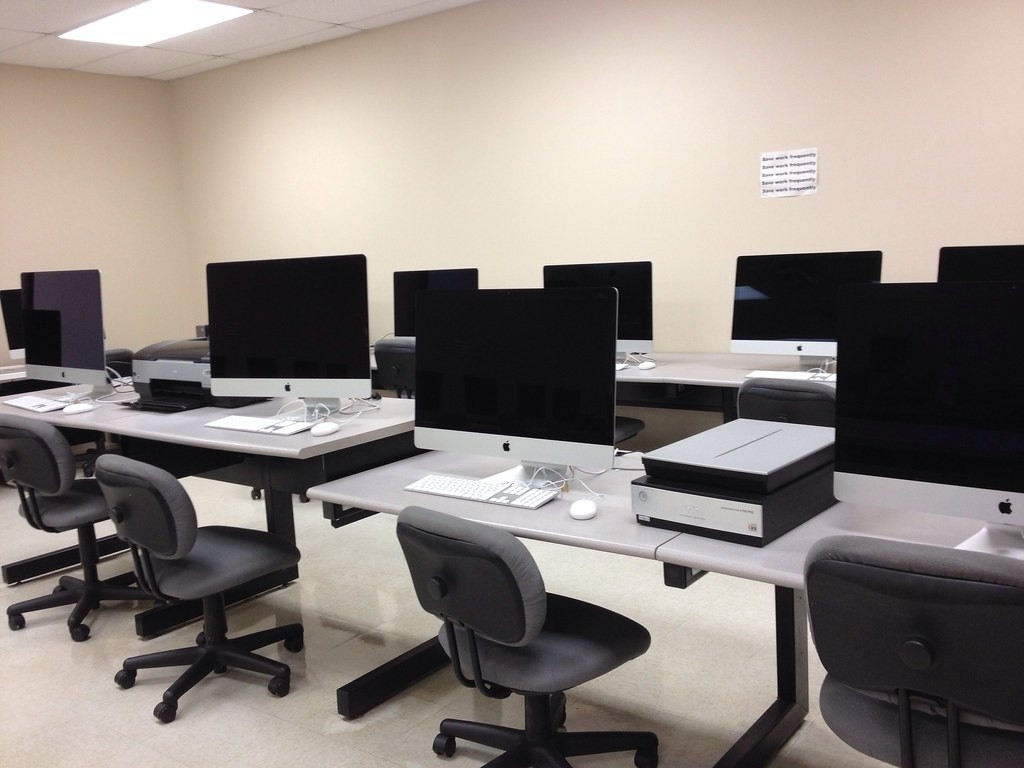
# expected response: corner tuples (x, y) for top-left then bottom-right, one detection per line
(737, 377), (836, 428)
(0, 414), (155, 640)
(374, 338), (415, 399)
(805, 536), (1024, 768)
(94, 453), (303, 723)
(615, 416), (644, 443)
(396, 505), (660, 768)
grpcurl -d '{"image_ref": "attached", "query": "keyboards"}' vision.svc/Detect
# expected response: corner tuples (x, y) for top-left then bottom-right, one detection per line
(4, 396), (68, 413)
(203, 415), (315, 436)
(616, 364), (629, 371)
(405, 475), (560, 510)
(745, 370), (837, 383)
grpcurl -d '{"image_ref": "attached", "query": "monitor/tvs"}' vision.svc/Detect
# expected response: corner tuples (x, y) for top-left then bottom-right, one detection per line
(0, 245), (1024, 560)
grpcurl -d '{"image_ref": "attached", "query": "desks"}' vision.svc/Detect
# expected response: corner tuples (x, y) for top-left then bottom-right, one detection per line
(306, 450), (1023, 768)
(0, 376), (415, 637)
(616, 353), (799, 423)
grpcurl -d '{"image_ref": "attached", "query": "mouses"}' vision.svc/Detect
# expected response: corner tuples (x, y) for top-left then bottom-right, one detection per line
(63, 404), (94, 414)
(311, 422), (340, 436)
(569, 499), (597, 520)
(639, 362), (656, 370)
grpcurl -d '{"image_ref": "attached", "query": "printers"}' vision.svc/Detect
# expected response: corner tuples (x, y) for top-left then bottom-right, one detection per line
(117, 325), (274, 413)
